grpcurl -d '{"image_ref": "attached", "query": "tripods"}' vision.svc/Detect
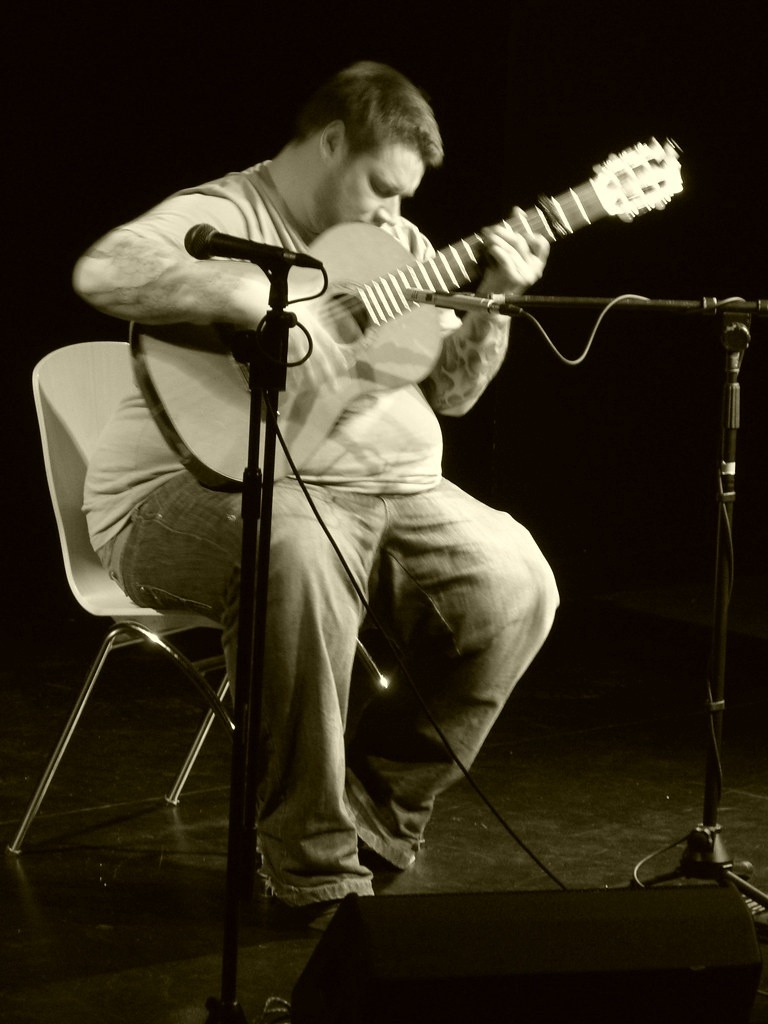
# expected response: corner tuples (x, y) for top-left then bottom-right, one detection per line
(435, 289), (768, 910)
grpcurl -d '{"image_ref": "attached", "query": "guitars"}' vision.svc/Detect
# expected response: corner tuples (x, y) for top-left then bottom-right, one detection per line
(130, 138), (682, 496)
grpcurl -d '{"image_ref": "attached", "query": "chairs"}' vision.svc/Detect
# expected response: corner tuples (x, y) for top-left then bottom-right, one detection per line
(6, 341), (394, 855)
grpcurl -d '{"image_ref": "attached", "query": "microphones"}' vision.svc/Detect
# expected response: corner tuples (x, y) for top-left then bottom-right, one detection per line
(184, 223), (324, 269)
(404, 288), (523, 317)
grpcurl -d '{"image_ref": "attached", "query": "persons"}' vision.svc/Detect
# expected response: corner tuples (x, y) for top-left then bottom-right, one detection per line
(75, 62), (560, 932)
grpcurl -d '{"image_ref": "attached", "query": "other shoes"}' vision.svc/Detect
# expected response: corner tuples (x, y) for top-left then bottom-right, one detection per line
(268, 890), (343, 931)
(361, 845), (402, 892)
